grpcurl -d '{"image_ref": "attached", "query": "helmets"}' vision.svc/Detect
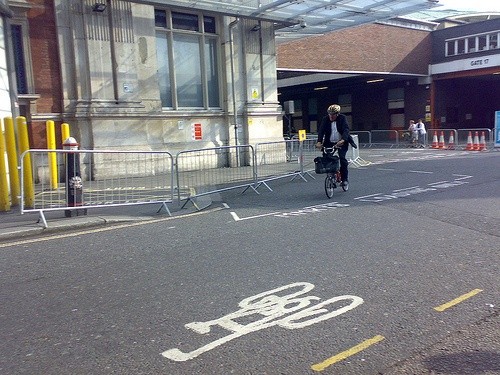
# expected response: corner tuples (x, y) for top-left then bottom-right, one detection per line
(327, 104), (340, 114)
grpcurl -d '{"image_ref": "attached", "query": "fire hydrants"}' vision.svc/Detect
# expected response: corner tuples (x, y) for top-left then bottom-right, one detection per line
(62, 137), (87, 217)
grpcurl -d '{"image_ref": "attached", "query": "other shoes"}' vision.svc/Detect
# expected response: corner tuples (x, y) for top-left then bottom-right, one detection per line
(342, 180), (348, 186)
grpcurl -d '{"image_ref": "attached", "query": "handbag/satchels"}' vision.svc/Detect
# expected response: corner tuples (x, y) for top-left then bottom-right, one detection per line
(314, 156), (340, 174)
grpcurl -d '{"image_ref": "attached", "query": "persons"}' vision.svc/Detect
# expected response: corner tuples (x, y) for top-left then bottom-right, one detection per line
(315, 104), (357, 187)
(408, 119), (427, 148)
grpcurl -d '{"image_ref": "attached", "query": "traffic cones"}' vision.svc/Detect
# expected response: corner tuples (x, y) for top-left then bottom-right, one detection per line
(438, 131), (445, 149)
(431, 131), (439, 149)
(479, 131), (486, 150)
(473, 131), (480, 150)
(466, 131), (473, 150)
(447, 131), (456, 146)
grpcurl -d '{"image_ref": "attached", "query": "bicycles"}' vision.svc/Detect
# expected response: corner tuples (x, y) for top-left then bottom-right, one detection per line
(314, 144), (350, 199)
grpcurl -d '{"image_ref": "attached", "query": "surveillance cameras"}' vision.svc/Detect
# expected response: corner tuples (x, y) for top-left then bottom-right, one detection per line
(300, 21), (306, 28)
